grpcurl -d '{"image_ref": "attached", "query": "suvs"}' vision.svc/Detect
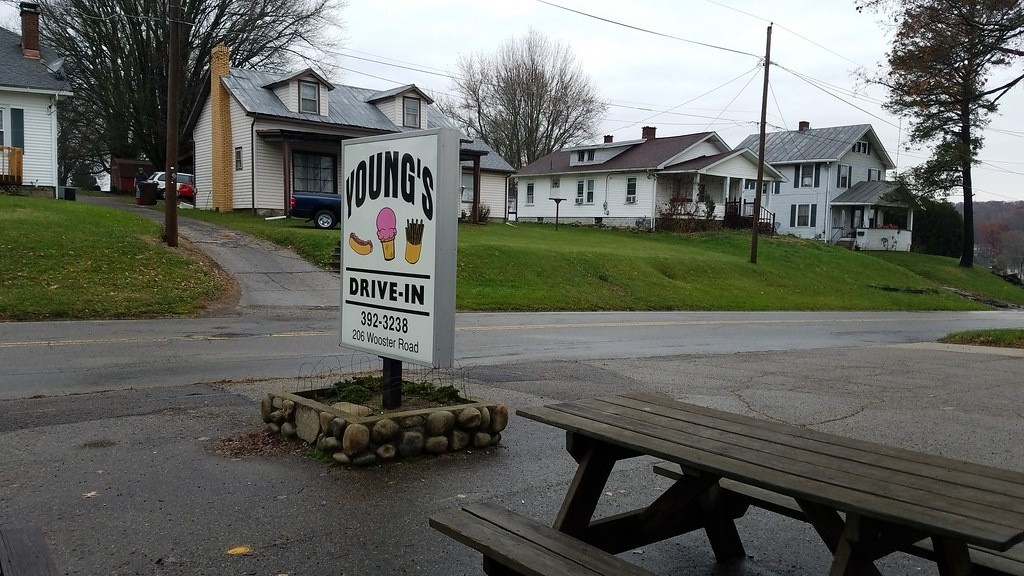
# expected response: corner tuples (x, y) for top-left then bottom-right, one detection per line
(147, 172), (194, 200)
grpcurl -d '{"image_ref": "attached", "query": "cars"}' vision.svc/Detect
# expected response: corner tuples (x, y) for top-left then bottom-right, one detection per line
(289, 193), (341, 229)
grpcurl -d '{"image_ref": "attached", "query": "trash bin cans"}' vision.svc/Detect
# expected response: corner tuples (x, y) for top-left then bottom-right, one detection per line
(137, 180), (160, 205)
(63, 187), (76, 201)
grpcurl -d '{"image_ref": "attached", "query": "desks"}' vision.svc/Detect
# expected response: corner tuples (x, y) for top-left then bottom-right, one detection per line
(516, 391), (1024, 576)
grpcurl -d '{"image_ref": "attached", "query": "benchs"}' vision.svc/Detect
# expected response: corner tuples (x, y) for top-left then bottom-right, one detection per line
(428, 501), (658, 576)
(653, 461), (1024, 576)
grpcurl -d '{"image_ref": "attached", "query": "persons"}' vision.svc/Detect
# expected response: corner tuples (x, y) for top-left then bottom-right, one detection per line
(134, 167), (148, 205)
